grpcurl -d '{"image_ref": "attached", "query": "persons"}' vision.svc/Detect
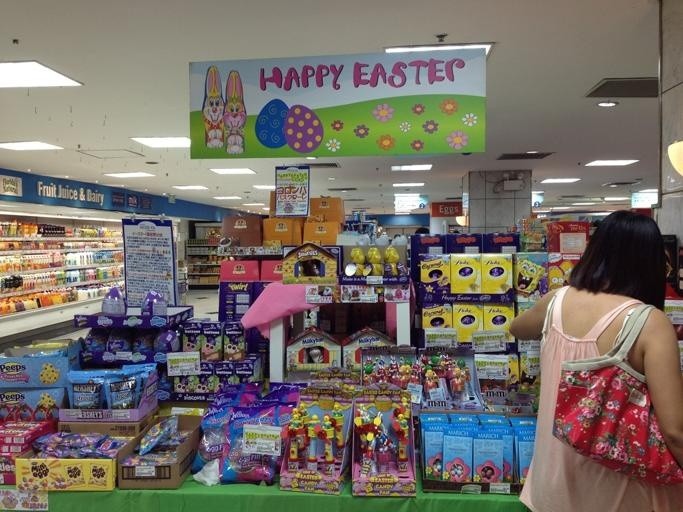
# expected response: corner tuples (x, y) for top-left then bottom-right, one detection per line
(506, 207), (683, 512)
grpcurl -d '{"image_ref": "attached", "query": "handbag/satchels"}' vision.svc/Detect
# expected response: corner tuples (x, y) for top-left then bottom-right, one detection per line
(553, 354), (683, 486)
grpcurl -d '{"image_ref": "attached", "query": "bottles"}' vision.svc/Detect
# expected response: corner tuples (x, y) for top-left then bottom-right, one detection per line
(0, 219), (100, 317)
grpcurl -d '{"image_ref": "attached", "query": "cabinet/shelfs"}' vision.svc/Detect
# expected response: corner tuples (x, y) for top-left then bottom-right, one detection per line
(0, 210), (221, 359)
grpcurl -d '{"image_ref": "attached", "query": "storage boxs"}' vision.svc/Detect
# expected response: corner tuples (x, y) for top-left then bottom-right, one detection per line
(1, 339), (203, 491)
